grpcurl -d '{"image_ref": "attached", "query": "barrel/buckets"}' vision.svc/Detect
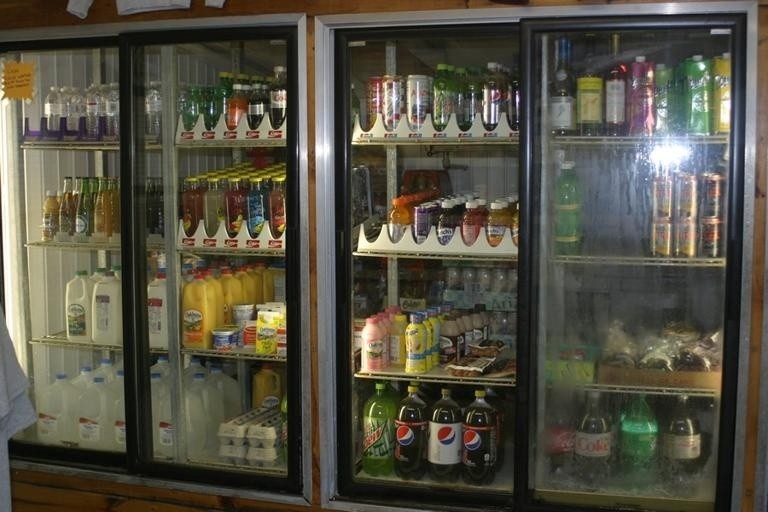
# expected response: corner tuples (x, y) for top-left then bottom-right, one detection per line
(64, 260), (274, 349)
(37, 357), (280, 460)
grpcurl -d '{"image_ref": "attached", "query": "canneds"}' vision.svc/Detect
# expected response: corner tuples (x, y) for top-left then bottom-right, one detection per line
(366, 73), (436, 134)
(442, 79), (523, 130)
(637, 169), (725, 264)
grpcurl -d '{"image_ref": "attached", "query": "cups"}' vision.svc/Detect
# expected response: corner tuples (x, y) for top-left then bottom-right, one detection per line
(208, 302), (257, 352)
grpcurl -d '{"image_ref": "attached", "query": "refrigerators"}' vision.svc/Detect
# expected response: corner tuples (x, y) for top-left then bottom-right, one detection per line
(305, 5), (749, 512)
(2, 17), (309, 508)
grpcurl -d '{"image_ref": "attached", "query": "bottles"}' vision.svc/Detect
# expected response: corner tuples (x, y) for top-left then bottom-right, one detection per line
(278, 392), (287, 472)
(552, 37), (626, 139)
(430, 61), (519, 134)
(547, 384), (709, 494)
(32, 67), (287, 246)
(359, 300), (491, 373)
(383, 189), (521, 250)
(357, 378), (508, 488)
(556, 160), (585, 256)
